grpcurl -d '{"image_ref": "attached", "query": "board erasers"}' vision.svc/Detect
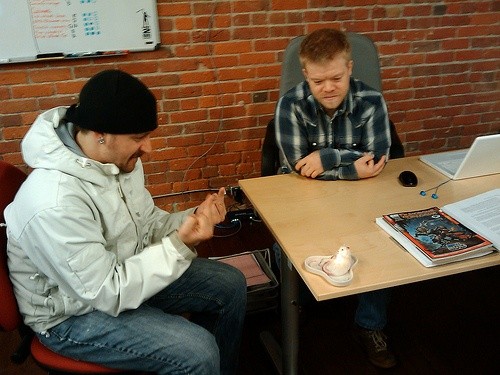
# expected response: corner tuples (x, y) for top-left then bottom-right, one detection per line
(37, 53), (64, 59)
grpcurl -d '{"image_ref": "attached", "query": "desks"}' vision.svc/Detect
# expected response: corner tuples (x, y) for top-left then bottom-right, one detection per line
(238, 149), (499, 375)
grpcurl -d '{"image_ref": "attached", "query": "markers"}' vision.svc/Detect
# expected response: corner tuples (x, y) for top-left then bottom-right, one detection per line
(66, 50), (128, 57)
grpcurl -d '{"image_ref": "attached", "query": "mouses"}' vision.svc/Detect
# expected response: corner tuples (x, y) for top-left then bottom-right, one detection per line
(399, 171), (417, 187)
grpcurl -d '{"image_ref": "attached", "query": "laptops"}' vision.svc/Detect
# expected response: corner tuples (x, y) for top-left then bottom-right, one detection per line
(419, 133), (500, 181)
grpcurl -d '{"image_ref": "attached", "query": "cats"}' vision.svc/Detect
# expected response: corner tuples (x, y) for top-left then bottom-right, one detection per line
(321, 244), (352, 276)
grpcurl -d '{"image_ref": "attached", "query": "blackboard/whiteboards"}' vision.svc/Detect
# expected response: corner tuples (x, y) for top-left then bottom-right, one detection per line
(0, 0), (161, 64)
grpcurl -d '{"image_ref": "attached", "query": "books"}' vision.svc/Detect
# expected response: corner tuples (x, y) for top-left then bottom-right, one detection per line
(376, 188), (500, 267)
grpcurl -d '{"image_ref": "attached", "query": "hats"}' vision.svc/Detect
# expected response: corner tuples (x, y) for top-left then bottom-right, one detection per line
(76, 69), (160, 136)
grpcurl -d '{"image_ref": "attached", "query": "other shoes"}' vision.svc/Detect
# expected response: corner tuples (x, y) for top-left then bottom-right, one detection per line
(350, 321), (397, 369)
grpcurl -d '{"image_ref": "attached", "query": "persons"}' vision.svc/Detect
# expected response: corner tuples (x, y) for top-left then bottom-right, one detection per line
(275, 28), (400, 369)
(4, 69), (247, 375)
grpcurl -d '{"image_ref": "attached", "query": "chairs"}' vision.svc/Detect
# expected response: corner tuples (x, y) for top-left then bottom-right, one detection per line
(260, 33), (405, 177)
(0, 159), (189, 375)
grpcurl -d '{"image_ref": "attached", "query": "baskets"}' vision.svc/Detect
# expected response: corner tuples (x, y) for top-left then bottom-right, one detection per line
(209, 248), (280, 313)
